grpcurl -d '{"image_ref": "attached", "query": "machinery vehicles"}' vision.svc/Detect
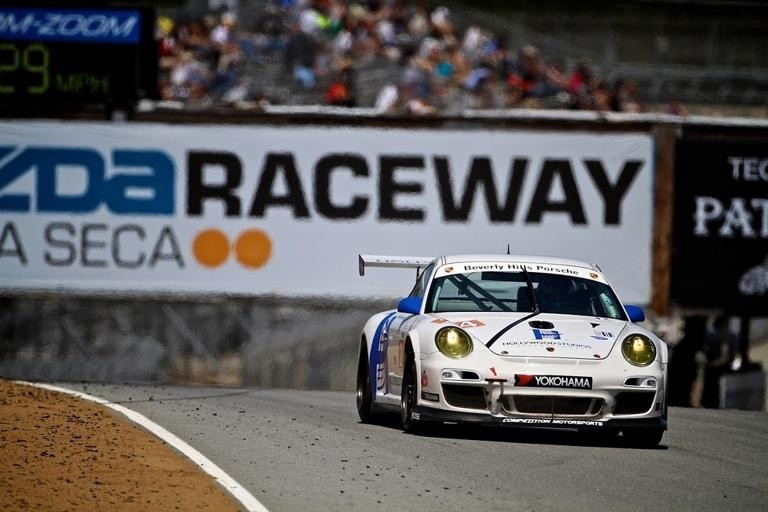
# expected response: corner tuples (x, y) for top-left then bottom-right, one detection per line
(345, 247), (672, 448)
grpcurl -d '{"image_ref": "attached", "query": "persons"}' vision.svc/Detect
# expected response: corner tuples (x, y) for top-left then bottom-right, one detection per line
(158, 0), (685, 115)
